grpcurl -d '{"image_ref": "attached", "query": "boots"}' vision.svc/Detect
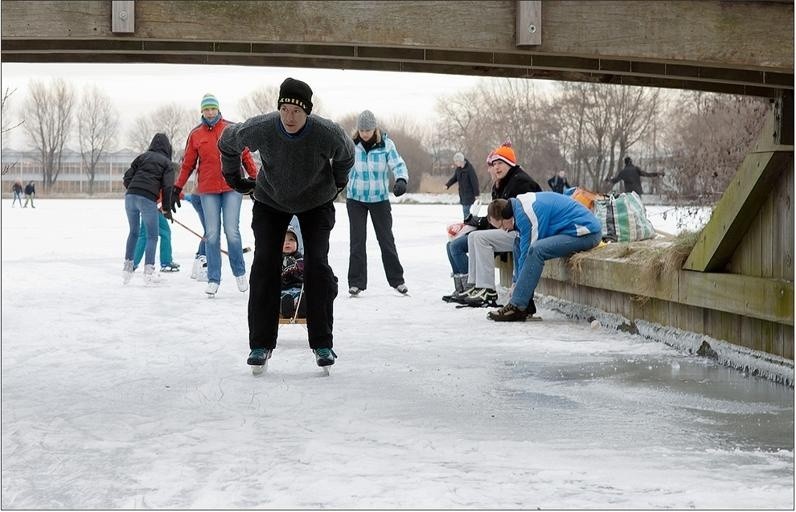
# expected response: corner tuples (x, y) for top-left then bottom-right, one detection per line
(442, 272), (472, 303)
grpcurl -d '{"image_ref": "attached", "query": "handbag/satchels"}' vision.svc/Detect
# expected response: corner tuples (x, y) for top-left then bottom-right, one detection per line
(572, 188), (658, 241)
(470, 199), (482, 216)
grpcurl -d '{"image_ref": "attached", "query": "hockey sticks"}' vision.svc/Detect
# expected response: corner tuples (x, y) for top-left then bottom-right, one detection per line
(172, 218), (250, 257)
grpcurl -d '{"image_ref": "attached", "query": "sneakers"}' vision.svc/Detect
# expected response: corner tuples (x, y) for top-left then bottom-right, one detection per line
(281, 290), (307, 319)
(348, 286), (362, 294)
(487, 300), (537, 322)
(459, 284), (499, 303)
(248, 347), (272, 366)
(311, 349), (338, 367)
(123, 254), (207, 282)
(397, 283), (409, 294)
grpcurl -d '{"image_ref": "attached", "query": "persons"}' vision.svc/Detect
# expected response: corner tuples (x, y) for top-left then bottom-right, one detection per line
(443, 150), (480, 220)
(121, 133), (173, 284)
(132, 160), (184, 270)
(276, 224), (315, 318)
(190, 167), (220, 272)
(606, 157), (668, 197)
(486, 186), (603, 329)
(438, 150), (498, 303)
(11, 179), (22, 207)
(545, 168), (569, 194)
(218, 76), (356, 365)
(454, 145), (542, 304)
(344, 108), (410, 295)
(172, 90), (259, 295)
(24, 180), (36, 208)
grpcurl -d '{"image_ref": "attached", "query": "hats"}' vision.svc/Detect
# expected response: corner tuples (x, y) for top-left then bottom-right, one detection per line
(357, 110), (376, 132)
(278, 77), (314, 116)
(487, 140), (517, 168)
(201, 93), (220, 111)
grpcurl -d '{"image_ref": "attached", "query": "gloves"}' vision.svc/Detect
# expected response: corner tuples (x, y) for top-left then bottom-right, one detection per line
(171, 185), (181, 214)
(222, 173), (256, 202)
(393, 179), (407, 197)
